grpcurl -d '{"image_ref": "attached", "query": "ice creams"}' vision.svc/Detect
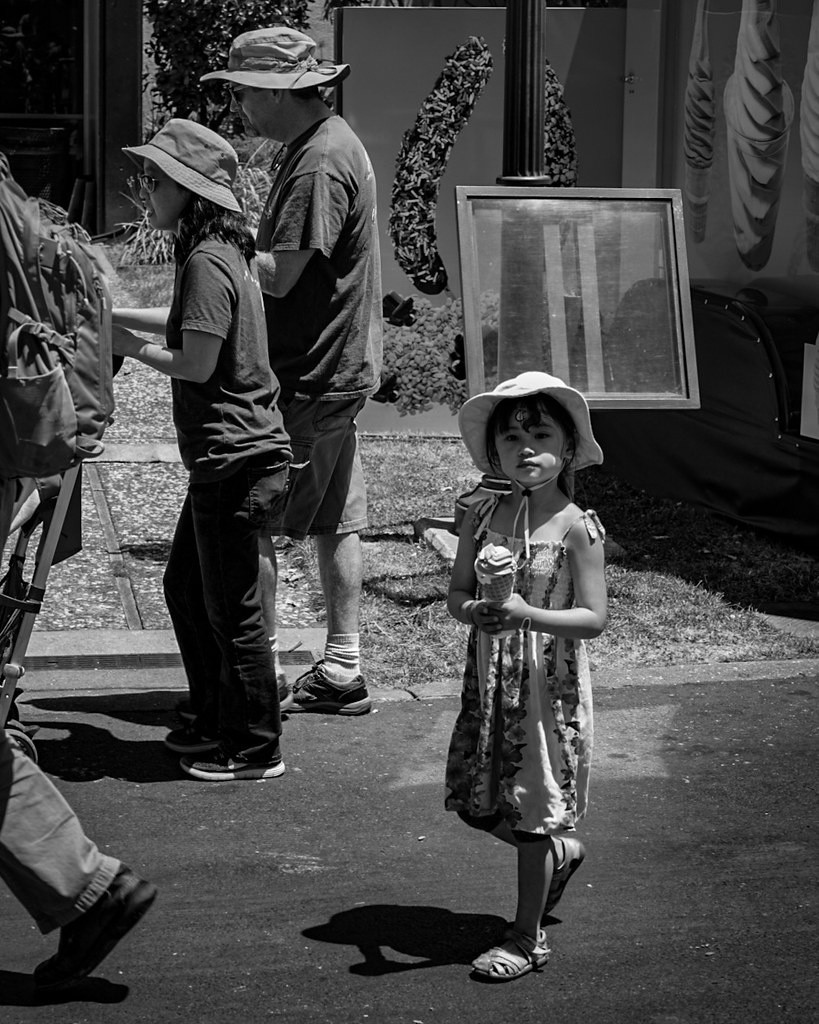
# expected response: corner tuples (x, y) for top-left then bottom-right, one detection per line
(474, 542), (517, 602)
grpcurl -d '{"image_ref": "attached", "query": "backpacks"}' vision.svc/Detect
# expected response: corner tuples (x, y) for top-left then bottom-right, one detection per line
(0, 176), (117, 480)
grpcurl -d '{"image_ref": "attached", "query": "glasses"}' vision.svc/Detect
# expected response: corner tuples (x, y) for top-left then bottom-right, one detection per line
(127, 175), (170, 193)
(222, 84), (250, 104)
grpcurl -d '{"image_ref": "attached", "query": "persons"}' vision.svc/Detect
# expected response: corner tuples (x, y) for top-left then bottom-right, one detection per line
(0, 151), (158, 993)
(112, 119), (294, 780)
(445, 371), (606, 979)
(38, 128), (87, 206)
(174, 27), (385, 720)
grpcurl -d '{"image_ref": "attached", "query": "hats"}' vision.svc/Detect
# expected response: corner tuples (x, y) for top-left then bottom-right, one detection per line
(458, 371), (603, 480)
(121, 118), (243, 214)
(199, 26), (351, 89)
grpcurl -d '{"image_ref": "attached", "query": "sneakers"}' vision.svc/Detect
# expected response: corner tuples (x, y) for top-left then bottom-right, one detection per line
(164, 720), (225, 753)
(179, 736), (286, 781)
(178, 672), (296, 720)
(283, 658), (372, 715)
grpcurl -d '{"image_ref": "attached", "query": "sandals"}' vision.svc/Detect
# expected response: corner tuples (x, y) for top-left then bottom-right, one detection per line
(471, 928), (553, 979)
(543, 836), (585, 915)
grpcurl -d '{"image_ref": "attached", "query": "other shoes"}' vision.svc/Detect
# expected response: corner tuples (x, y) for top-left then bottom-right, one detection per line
(32, 861), (158, 994)
